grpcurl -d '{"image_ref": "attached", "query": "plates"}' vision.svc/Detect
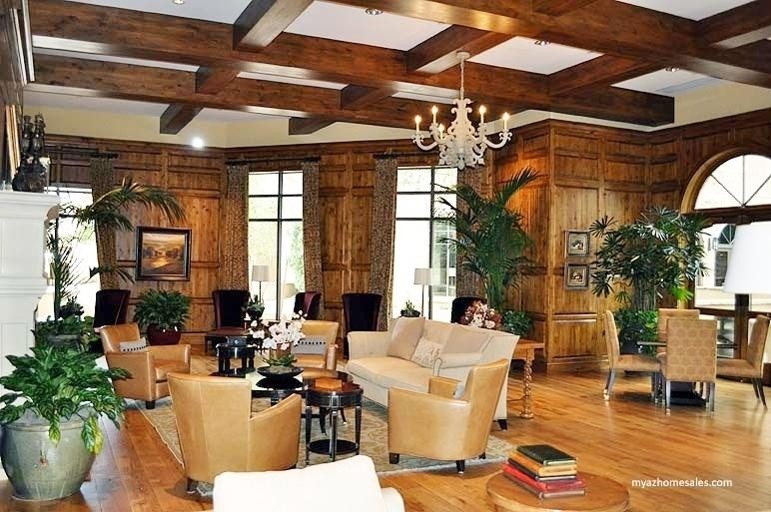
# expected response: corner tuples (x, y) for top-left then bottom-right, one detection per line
(256, 379), (304, 390)
(257, 366), (304, 379)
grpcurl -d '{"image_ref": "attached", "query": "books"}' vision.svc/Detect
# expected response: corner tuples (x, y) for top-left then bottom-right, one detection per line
(502, 442), (585, 498)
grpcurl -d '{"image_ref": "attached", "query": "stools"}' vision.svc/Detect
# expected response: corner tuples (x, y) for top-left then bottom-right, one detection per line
(301, 407), (348, 436)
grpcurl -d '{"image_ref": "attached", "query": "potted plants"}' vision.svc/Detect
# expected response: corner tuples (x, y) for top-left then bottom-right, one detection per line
(588, 202), (713, 378)
(33, 313), (102, 349)
(1, 342), (133, 500)
(240, 294), (265, 319)
(60, 295), (84, 320)
(400, 299), (420, 317)
(132, 288), (192, 346)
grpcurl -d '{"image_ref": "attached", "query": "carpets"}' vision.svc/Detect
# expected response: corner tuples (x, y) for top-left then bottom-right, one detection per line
(133, 396), (515, 497)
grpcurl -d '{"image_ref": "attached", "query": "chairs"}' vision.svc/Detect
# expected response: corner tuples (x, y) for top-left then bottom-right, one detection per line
(93, 289), (131, 351)
(603, 309), (661, 403)
(342, 293), (382, 357)
(716, 314), (770, 407)
(292, 291), (322, 325)
(657, 307), (700, 353)
(212, 290), (250, 329)
(661, 319), (717, 412)
(451, 297), (487, 324)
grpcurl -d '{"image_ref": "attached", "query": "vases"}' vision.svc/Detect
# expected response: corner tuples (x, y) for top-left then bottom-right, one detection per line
(258, 366), (304, 384)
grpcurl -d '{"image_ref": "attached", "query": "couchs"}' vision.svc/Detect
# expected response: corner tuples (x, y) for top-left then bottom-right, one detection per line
(288, 320), (340, 369)
(387, 358), (509, 474)
(347, 316), (521, 430)
(99, 322), (192, 410)
(212, 454), (405, 512)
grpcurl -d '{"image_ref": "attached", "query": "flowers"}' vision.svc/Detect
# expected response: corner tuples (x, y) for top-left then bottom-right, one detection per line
(246, 310), (308, 366)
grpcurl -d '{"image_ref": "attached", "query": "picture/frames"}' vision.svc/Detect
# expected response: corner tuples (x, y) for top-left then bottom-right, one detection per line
(563, 262), (591, 291)
(135, 226), (192, 283)
(564, 228), (592, 259)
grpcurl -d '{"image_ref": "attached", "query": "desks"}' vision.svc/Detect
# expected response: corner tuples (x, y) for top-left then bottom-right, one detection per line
(511, 338), (545, 419)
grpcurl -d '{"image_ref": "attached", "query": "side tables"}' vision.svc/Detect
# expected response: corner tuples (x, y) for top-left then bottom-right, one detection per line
(486, 473), (631, 512)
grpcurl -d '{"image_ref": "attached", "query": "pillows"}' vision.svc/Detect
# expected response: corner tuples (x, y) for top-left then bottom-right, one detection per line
(440, 326), (488, 359)
(387, 316), (426, 360)
(411, 336), (444, 369)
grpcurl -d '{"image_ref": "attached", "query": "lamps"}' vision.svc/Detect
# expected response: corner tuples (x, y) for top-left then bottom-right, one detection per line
(413, 267), (441, 317)
(252, 265), (270, 303)
(411, 51), (513, 170)
